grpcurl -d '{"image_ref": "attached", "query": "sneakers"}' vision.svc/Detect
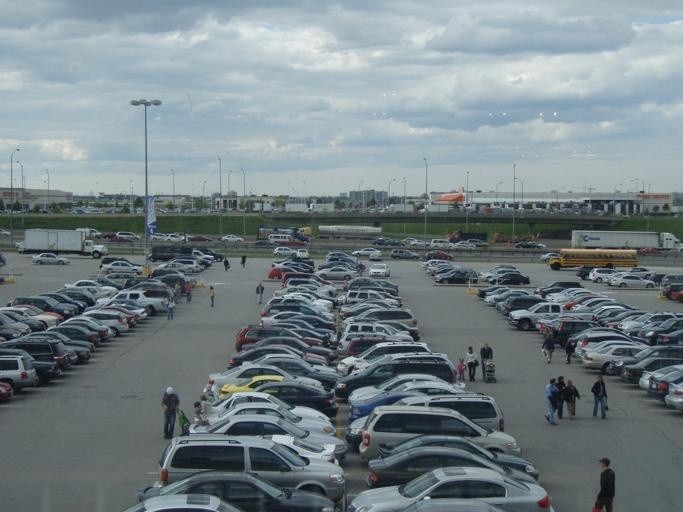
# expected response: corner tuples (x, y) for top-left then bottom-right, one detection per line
(544, 414), (577, 426)
(163, 432), (174, 438)
(458, 378), (475, 382)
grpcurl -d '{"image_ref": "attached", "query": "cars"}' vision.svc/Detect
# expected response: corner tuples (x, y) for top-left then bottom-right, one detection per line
(0, 225), (123, 421)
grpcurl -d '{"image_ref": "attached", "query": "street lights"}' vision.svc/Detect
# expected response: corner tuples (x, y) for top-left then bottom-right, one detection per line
(91, 156), (253, 216)
(128, 98), (163, 279)
(5, 142), (59, 217)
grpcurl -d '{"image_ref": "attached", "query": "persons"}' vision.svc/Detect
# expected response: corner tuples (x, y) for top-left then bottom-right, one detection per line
(241, 255), (246, 268)
(167, 297), (176, 320)
(256, 282), (264, 304)
(173, 282), (181, 301)
(542, 331), (556, 363)
(199, 393), (210, 412)
(457, 343), (494, 381)
(206, 380), (218, 402)
(194, 401), (203, 424)
(185, 279), (192, 302)
(208, 286), (216, 306)
(565, 334), (575, 365)
(593, 458), (615, 512)
(544, 376), (610, 425)
(223, 257), (230, 272)
(162, 387), (180, 440)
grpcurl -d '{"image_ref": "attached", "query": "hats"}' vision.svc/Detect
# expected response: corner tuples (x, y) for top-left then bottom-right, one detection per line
(166, 387), (174, 395)
(598, 458), (610, 465)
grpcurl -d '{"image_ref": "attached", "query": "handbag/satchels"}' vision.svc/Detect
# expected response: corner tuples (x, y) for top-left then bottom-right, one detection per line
(167, 302), (169, 307)
(603, 394), (608, 411)
(473, 359), (479, 366)
(593, 501), (604, 512)
